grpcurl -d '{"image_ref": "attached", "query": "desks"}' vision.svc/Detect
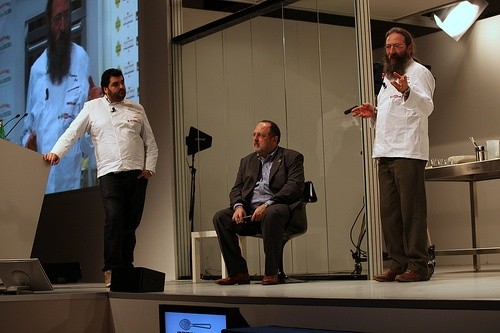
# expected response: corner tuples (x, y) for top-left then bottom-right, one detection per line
(422, 158), (499, 275)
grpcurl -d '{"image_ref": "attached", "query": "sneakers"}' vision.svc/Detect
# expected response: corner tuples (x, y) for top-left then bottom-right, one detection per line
(373, 269), (425, 282)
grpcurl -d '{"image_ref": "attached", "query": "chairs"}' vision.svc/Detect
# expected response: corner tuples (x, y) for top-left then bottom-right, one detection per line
(238, 179), (318, 285)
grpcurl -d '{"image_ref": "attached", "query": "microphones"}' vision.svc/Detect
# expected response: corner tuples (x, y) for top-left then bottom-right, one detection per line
(2, 113), (28, 139)
(3, 114), (21, 127)
(344, 105), (358, 115)
(382, 81), (387, 89)
(111, 106), (116, 113)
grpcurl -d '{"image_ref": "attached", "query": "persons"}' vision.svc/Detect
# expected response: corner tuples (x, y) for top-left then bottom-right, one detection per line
(42, 69), (158, 291)
(18, 0), (104, 195)
(212, 120), (305, 285)
(351, 27), (436, 283)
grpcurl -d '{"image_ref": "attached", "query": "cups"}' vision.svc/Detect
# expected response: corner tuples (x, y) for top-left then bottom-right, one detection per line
(426, 159), (448, 166)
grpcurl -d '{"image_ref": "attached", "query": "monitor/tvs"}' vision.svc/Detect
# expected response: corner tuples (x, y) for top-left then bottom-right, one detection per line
(158, 304), (240, 333)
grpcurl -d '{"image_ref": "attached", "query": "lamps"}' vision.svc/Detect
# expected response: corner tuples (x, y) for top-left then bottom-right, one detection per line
(431, 0), (490, 43)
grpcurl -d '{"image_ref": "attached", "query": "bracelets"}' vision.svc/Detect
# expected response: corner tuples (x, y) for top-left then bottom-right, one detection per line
(265, 203), (269, 208)
(372, 109), (377, 119)
(149, 171), (155, 177)
(403, 87), (410, 97)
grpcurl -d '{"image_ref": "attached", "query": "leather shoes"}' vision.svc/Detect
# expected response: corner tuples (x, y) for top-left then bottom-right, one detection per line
(215, 274), (279, 285)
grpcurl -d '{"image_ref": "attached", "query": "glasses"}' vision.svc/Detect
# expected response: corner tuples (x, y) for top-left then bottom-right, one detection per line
(49, 10), (72, 24)
(384, 43), (406, 49)
